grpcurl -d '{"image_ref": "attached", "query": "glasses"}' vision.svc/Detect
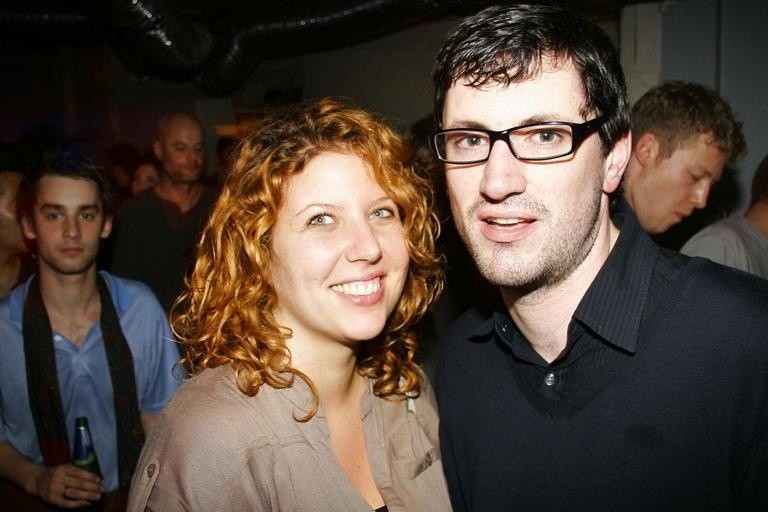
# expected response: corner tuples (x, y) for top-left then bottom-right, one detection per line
(426, 105), (624, 167)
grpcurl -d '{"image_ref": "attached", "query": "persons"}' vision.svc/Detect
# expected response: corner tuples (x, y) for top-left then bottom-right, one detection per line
(0, 163), (36, 301)
(679, 157), (768, 280)
(395, 12), (768, 512)
(0, 160), (189, 512)
(127, 97), (454, 512)
(111, 110), (223, 320)
(621, 82), (749, 252)
(127, 160), (167, 194)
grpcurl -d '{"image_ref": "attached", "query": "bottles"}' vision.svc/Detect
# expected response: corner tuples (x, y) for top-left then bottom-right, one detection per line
(74, 415), (104, 510)
